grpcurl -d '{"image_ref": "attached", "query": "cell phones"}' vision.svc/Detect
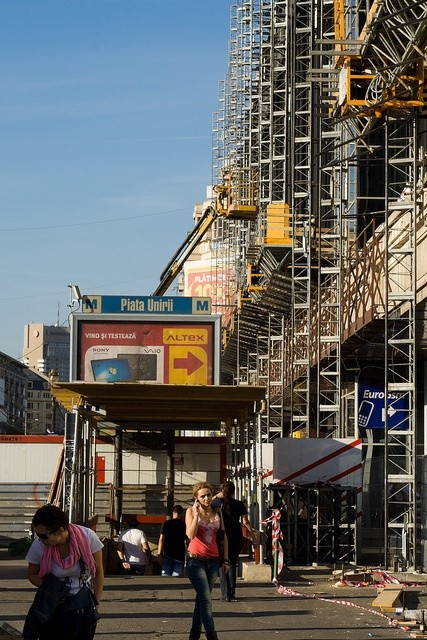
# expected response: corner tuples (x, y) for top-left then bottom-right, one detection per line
(358, 400), (374, 428)
(195, 499), (199, 504)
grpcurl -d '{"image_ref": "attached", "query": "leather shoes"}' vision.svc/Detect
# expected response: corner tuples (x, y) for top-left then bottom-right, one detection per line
(221, 598), (230, 601)
(229, 596), (235, 600)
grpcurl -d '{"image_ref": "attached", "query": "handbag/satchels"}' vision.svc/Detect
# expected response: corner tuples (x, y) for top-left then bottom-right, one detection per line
(216, 530), (224, 543)
(60, 586), (102, 636)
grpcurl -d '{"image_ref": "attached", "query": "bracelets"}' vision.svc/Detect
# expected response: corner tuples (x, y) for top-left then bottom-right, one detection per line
(214, 495), (217, 499)
(223, 559), (231, 565)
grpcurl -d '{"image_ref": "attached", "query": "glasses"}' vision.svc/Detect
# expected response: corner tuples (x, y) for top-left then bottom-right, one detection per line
(34, 527), (63, 539)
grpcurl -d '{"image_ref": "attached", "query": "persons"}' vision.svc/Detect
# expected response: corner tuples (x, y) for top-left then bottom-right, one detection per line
(156, 505), (187, 576)
(116, 519), (153, 575)
(211, 482), (257, 601)
(183, 481), (231, 640)
(26, 504), (104, 640)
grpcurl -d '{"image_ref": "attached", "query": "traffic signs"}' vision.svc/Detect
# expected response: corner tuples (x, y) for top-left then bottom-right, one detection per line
(82, 295), (211, 316)
